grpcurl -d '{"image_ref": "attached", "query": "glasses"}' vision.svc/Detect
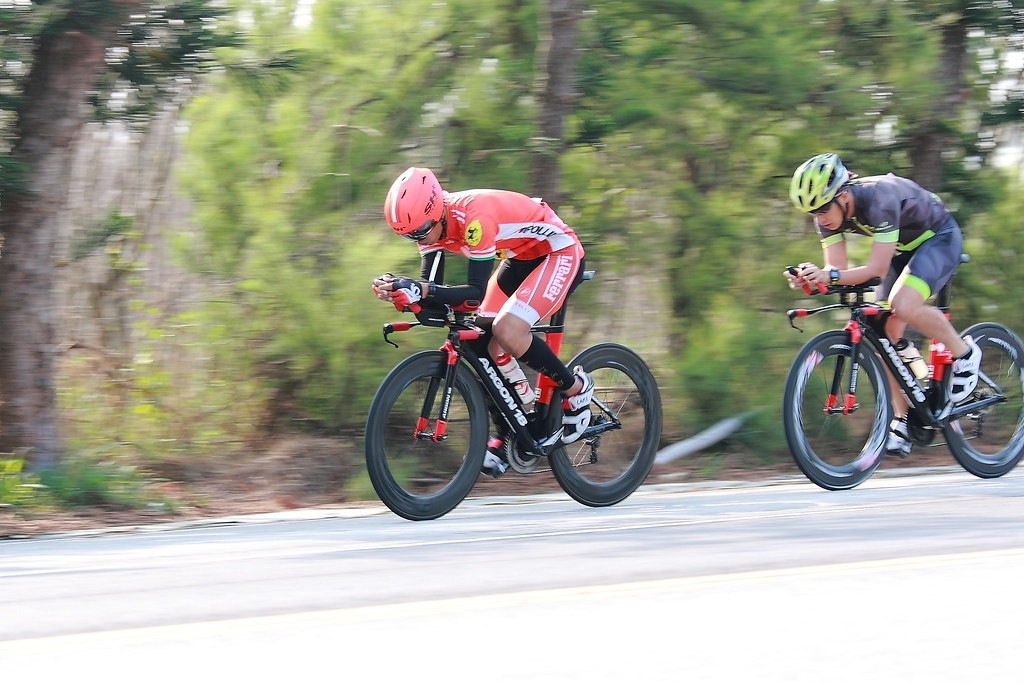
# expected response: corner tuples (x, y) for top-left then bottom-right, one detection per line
(808, 191), (843, 216)
(399, 220), (437, 242)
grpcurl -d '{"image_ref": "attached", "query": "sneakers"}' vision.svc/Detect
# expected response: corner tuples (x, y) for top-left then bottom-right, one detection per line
(561, 366), (594, 445)
(462, 435), (512, 480)
(947, 335), (983, 403)
(885, 417), (912, 458)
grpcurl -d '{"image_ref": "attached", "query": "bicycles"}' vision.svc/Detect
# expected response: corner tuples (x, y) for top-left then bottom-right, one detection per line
(782, 252), (1024, 492)
(363, 270), (664, 522)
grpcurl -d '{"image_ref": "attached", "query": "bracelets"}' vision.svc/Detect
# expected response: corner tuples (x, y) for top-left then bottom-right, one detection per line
(423, 280), (436, 304)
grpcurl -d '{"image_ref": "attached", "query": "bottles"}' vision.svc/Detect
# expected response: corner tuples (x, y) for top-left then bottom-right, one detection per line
(895, 339), (928, 380)
(496, 352), (535, 404)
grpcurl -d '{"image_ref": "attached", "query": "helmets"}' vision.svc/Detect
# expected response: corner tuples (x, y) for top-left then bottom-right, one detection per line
(383, 166), (444, 242)
(788, 152), (851, 213)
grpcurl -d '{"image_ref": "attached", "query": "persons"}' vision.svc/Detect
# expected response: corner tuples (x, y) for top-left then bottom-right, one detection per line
(784, 152), (982, 451)
(372, 166), (596, 476)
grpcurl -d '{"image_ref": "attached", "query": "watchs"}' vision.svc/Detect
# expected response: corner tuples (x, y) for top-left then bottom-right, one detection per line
(826, 268), (840, 285)
